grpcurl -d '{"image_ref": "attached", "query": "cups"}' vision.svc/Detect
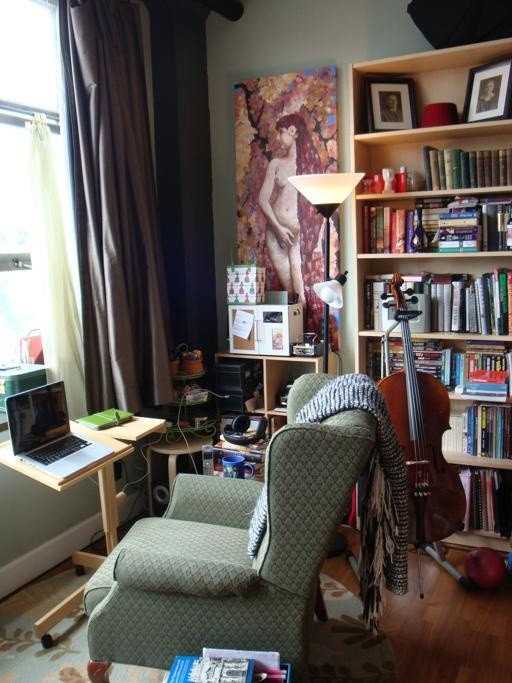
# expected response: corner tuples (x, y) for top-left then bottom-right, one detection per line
(222, 455), (253, 479)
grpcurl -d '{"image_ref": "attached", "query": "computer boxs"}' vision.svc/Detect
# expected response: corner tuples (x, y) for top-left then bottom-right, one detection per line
(211, 358), (262, 415)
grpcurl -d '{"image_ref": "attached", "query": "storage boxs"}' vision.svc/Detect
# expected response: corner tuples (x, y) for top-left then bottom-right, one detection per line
(0, 364), (47, 413)
(228, 304), (303, 357)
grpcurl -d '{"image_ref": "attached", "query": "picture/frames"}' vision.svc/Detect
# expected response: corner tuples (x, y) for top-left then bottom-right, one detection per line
(460, 54), (512, 123)
(363, 73), (417, 133)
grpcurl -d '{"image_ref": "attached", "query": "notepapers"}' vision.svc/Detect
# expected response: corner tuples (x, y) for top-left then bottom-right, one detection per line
(232, 310), (254, 339)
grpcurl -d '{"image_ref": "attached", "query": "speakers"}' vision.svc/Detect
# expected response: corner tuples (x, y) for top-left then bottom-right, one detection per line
(150, 455), (189, 517)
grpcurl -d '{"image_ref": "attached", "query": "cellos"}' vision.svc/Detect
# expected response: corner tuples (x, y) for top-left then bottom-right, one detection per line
(376, 274), (467, 545)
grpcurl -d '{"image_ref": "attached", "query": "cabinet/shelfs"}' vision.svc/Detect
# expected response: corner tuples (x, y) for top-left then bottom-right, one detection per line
(214, 351), (338, 482)
(347, 38), (512, 558)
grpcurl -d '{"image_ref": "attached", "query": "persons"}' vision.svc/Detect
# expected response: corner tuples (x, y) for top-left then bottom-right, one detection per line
(251, 112), (318, 312)
(413, 198), (512, 252)
(478, 75), (499, 112)
(379, 90), (403, 122)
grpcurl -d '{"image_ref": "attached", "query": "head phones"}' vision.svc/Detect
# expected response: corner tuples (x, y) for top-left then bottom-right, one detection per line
(222, 414), (267, 446)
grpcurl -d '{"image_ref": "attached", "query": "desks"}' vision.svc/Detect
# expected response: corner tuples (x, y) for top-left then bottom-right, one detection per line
(0, 415), (166, 649)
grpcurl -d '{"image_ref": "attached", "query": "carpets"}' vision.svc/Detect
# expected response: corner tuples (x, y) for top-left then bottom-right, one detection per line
(0, 566), (397, 683)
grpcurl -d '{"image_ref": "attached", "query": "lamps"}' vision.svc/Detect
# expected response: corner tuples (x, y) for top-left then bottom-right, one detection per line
(313, 270), (349, 309)
(286, 172), (366, 623)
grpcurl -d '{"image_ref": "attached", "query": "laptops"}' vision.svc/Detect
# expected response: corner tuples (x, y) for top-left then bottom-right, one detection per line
(4, 381), (115, 480)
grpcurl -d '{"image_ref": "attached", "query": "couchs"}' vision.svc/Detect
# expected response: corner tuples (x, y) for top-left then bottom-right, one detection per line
(82, 372), (378, 683)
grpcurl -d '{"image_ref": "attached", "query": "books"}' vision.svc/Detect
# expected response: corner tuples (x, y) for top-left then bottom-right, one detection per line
(363, 205), (413, 253)
(365, 272), (452, 332)
(450, 342), (511, 404)
(167, 656), (256, 683)
(452, 268), (512, 338)
(444, 406), (512, 461)
(458, 469), (511, 539)
(422, 145), (512, 190)
(78, 408), (133, 431)
(366, 339), (454, 389)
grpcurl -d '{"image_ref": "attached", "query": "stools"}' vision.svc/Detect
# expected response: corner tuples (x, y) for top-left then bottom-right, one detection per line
(147, 435), (213, 516)
